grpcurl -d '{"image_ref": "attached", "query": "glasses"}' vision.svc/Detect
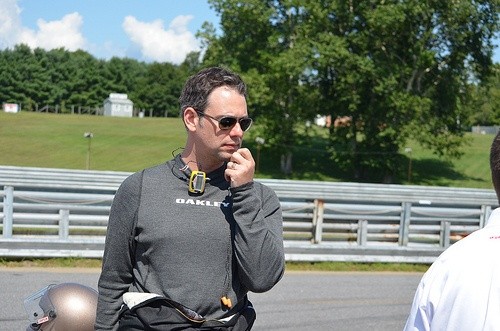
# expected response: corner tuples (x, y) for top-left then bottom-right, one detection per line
(198, 110), (252, 132)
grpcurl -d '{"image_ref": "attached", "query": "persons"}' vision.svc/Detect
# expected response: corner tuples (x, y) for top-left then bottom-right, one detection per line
(94, 65), (283, 331)
(404, 132), (499, 331)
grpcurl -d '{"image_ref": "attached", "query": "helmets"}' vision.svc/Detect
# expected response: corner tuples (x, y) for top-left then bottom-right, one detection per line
(28, 283), (98, 331)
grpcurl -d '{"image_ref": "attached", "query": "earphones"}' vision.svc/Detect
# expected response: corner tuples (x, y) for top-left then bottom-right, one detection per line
(221, 296), (232, 308)
